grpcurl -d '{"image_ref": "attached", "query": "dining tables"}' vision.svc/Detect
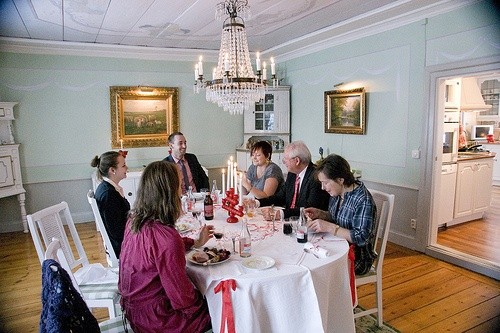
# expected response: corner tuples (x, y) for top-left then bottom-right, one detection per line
(171, 191), (356, 333)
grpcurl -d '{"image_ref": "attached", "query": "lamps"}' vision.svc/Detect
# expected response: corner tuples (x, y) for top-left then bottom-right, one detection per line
(193, 0), (276, 115)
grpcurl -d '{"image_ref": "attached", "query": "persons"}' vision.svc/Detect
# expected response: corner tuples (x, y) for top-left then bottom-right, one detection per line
(237, 140), (284, 207)
(257, 142), (330, 221)
(91, 150), (131, 259)
(161, 131), (210, 197)
(303, 154), (378, 274)
(119, 161), (212, 333)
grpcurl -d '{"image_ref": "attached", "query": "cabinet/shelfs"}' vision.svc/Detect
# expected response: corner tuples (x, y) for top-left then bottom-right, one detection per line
(243, 85), (290, 135)
(453, 157), (494, 219)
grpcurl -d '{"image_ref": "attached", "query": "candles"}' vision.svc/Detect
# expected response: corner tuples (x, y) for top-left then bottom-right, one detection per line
(221, 155), (244, 206)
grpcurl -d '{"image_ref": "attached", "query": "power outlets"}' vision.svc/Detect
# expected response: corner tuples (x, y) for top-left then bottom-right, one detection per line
(410, 218), (417, 230)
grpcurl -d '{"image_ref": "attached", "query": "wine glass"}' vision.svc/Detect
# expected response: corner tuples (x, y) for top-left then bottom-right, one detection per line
(246, 202), (256, 222)
(200, 188), (208, 204)
(213, 224), (223, 250)
(227, 224), (239, 255)
(268, 210), (278, 233)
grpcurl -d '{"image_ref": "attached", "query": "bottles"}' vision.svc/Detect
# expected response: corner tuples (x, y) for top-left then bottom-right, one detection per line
(296, 207), (308, 243)
(239, 218), (252, 257)
(204, 190), (213, 220)
(211, 179), (219, 204)
(283, 204), (292, 234)
(187, 185), (195, 212)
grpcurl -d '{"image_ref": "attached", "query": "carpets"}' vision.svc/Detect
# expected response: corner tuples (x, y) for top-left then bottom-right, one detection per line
(352, 304), (403, 333)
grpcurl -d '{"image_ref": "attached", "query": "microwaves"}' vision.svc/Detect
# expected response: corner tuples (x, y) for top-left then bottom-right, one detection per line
(470, 125), (494, 141)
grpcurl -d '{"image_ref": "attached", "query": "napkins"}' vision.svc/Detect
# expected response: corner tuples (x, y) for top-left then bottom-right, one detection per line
(186, 258), (242, 300)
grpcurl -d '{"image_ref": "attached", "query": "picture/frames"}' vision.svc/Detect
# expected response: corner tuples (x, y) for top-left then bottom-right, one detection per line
(324, 88), (366, 135)
(110, 86), (181, 148)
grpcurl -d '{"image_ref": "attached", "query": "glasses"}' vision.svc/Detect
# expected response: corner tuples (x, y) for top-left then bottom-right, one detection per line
(282, 156), (297, 163)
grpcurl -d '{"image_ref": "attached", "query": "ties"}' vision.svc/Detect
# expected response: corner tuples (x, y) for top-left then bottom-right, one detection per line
(178, 159), (191, 193)
(289, 174), (300, 208)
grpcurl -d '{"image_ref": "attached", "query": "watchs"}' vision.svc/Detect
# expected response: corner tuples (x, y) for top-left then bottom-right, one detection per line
(334, 225), (340, 236)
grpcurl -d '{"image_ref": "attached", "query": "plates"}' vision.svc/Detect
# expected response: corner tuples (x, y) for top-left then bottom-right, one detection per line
(187, 249), (231, 266)
(176, 222), (194, 233)
(242, 256), (275, 270)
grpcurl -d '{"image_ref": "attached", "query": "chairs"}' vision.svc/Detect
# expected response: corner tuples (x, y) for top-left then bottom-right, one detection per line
(354, 187), (394, 328)
(44, 238), (136, 333)
(27, 202), (124, 319)
(87, 190), (121, 275)
